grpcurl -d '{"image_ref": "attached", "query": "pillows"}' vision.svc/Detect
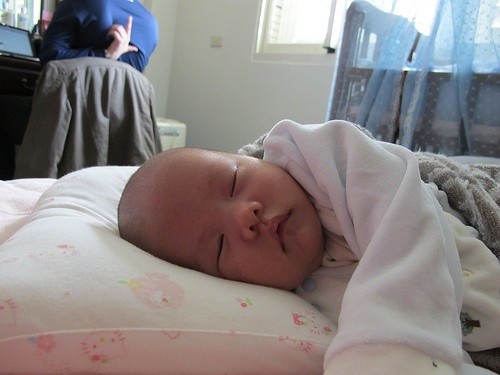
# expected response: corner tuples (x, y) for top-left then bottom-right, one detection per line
(0, 166), (337, 375)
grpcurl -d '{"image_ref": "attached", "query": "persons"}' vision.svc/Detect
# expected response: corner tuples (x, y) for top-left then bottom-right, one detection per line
(116, 117), (499, 373)
(37, 0), (160, 73)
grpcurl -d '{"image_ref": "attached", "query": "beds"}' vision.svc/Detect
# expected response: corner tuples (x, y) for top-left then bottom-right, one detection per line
(327, 0), (499, 158)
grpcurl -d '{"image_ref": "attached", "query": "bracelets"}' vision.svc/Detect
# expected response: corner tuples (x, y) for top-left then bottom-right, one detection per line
(104, 49), (112, 58)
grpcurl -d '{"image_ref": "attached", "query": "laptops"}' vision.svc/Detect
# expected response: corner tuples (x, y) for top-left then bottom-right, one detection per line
(0, 23), (40, 62)
